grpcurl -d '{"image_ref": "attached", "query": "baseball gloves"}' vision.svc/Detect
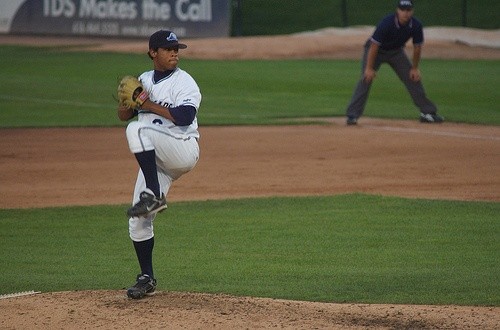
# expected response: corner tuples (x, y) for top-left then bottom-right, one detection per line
(113, 74), (150, 111)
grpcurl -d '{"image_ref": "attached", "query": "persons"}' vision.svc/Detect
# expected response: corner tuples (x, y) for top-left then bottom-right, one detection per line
(344, 1), (445, 125)
(116, 31), (202, 299)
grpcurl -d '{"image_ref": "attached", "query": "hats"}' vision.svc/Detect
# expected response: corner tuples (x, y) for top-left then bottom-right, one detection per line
(397, 0), (415, 8)
(149, 30), (187, 50)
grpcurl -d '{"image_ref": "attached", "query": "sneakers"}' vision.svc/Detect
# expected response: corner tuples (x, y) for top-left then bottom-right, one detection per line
(126, 188), (168, 219)
(126, 274), (157, 299)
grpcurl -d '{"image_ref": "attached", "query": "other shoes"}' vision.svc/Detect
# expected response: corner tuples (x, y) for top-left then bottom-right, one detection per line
(419, 112), (446, 123)
(347, 116), (358, 125)
